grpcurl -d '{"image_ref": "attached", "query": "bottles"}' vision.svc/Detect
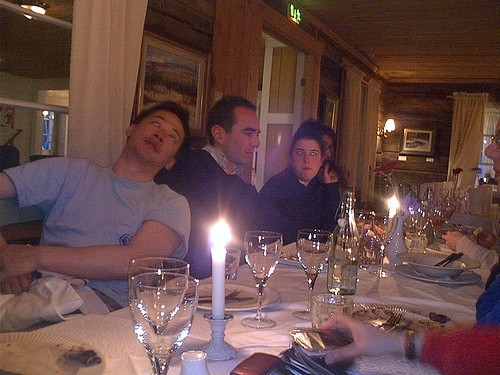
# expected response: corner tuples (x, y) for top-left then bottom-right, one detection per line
(326, 189), (359, 295)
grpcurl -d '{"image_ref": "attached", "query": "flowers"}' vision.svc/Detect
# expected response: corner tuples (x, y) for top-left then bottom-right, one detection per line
(370, 160), (400, 185)
(453, 168), (464, 190)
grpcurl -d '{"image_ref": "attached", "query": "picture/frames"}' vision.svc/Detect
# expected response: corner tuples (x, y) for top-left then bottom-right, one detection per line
(132, 30), (213, 139)
(399, 127), (434, 156)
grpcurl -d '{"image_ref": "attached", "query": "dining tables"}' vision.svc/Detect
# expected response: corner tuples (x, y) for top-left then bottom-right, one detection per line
(0, 199), (500, 375)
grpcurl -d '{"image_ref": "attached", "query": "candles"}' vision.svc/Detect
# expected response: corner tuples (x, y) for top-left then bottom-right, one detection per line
(209, 218), (232, 320)
(387, 194), (399, 218)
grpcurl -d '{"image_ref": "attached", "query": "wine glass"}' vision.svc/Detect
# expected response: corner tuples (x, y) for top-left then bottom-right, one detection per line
(244, 231), (282, 329)
(129, 272), (200, 375)
(369, 205), (398, 279)
(292, 228), (332, 321)
(128, 258), (189, 336)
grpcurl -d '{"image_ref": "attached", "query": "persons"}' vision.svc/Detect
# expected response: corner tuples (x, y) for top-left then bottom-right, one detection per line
(257, 120), (342, 258)
(0, 101), (192, 333)
(319, 311), (500, 375)
(442, 87), (500, 326)
(167, 94), (284, 279)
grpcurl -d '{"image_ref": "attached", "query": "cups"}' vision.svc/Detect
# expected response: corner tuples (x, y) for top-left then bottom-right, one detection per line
(311, 294), (353, 328)
(354, 187), (467, 265)
(225, 248), (242, 280)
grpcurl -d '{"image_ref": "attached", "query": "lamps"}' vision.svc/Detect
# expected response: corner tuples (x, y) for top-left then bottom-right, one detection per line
(376, 118), (395, 136)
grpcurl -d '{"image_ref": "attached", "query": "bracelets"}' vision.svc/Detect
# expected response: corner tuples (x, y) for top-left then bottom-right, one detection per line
(404, 329), (415, 359)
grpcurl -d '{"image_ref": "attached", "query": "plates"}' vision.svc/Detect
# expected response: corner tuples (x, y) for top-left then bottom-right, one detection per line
(395, 252), (481, 270)
(395, 265), (481, 284)
(425, 243), (455, 254)
(341, 303), (455, 332)
(258, 238), (329, 270)
(184, 284), (281, 312)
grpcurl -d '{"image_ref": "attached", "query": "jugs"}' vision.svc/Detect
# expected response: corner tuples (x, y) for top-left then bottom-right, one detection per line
(469, 184), (493, 214)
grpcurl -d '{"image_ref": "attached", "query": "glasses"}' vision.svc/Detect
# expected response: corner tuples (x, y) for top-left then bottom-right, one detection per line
(321, 142), (335, 153)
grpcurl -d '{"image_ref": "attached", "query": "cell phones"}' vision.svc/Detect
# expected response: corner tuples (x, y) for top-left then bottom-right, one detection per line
(290, 327), (353, 360)
